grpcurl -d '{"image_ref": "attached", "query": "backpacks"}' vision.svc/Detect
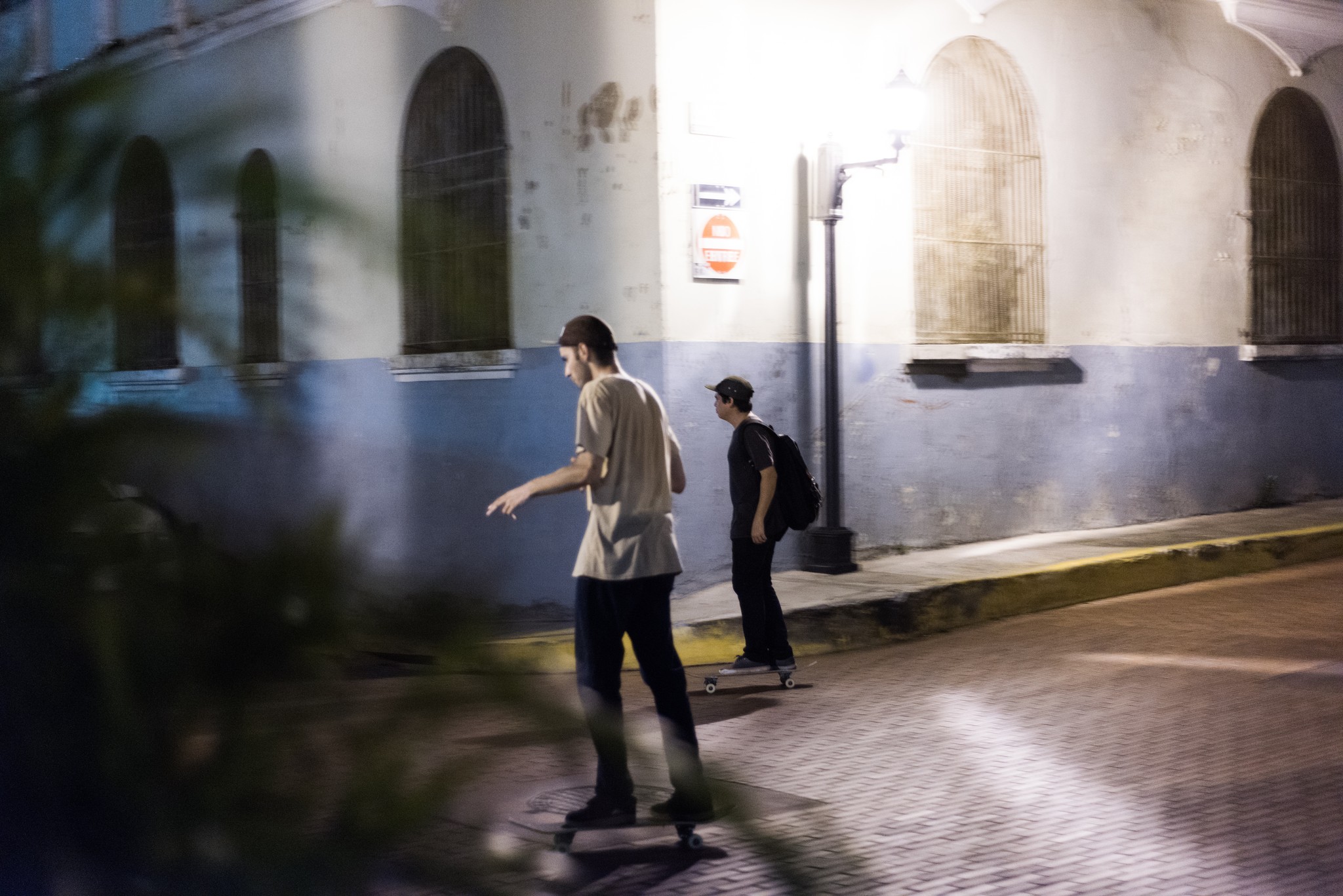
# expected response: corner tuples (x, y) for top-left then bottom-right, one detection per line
(738, 419), (821, 531)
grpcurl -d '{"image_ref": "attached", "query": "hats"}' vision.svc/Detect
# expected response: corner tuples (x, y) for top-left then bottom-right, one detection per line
(705, 376), (754, 402)
(542, 315), (617, 349)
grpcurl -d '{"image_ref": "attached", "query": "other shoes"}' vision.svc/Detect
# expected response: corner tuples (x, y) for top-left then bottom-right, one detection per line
(565, 796), (638, 827)
(649, 791), (714, 822)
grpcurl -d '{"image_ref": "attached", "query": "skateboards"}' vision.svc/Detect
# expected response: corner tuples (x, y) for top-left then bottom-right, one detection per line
(683, 660), (818, 693)
(521, 815), (721, 854)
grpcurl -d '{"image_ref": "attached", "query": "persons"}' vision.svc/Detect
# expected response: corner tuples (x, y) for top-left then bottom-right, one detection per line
(484, 316), (716, 830)
(704, 376), (824, 674)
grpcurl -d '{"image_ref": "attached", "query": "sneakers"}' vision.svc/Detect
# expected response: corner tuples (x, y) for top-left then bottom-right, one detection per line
(768, 657), (796, 670)
(719, 654), (770, 674)
